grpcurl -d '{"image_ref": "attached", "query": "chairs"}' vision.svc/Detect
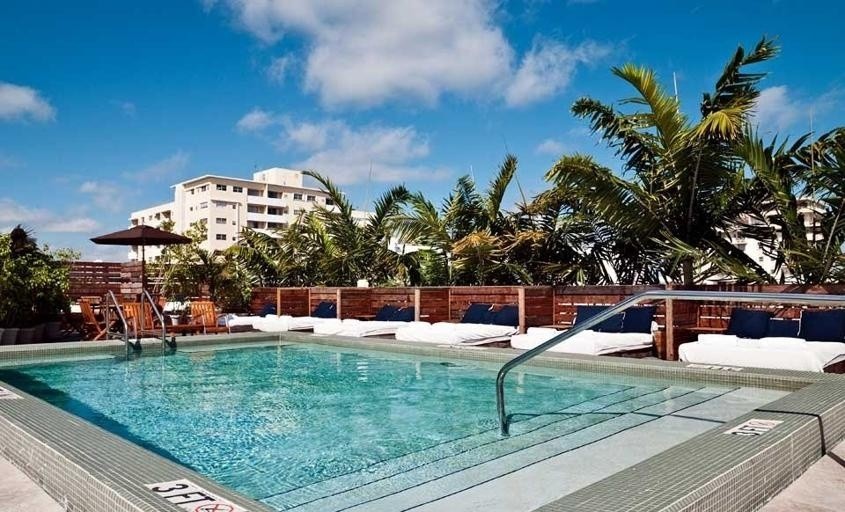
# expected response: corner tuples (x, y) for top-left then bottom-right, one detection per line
(64, 296), (845, 373)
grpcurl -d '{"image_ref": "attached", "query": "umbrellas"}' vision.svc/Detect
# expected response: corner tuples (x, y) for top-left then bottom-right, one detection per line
(90, 224), (191, 295)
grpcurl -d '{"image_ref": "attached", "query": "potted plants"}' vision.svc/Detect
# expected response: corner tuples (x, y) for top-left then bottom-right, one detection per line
(0, 235), (80, 345)
